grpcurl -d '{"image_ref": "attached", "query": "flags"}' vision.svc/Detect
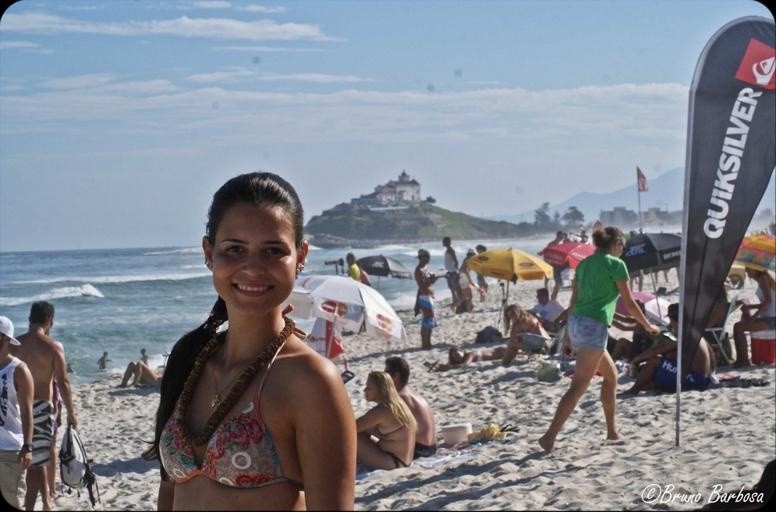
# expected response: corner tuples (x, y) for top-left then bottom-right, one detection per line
(636, 167), (650, 195)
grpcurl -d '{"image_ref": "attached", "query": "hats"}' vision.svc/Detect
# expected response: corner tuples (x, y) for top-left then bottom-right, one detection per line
(664, 303), (688, 317)
(746, 255), (768, 272)
(0, 315), (21, 346)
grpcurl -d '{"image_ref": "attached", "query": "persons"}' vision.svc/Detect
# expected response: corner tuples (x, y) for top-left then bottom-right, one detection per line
(1, 172), (776, 510)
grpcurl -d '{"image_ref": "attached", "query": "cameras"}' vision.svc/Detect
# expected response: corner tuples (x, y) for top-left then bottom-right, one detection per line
(325, 258), (343, 266)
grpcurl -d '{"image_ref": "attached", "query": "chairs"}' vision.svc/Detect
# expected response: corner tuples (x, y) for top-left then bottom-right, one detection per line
(518, 333), (548, 363)
(705, 295), (743, 368)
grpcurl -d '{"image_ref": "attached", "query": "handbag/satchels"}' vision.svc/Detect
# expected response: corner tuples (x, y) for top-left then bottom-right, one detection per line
(58, 424), (95, 489)
